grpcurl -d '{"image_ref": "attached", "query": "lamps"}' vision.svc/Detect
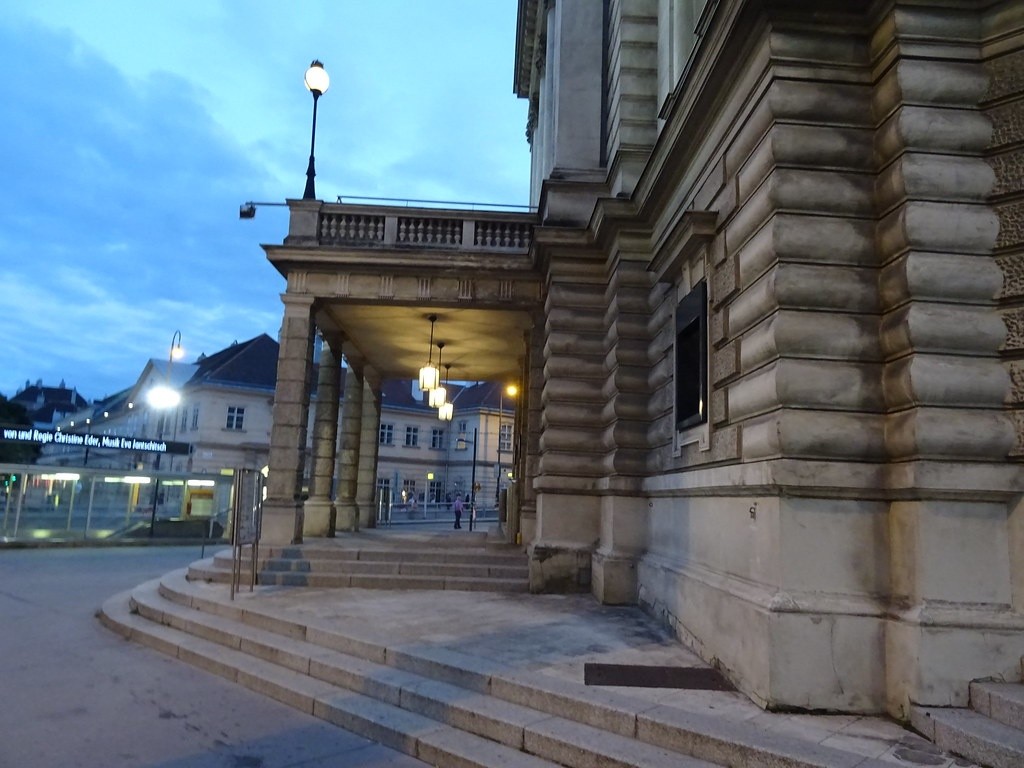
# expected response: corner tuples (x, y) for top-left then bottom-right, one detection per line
(429, 341), (447, 408)
(438, 363), (453, 421)
(239, 201), (288, 219)
(304, 57), (332, 96)
(419, 314), (440, 391)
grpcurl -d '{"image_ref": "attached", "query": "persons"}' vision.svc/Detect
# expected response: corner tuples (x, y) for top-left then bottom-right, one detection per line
(454, 496), (464, 529)
(446, 493), (453, 510)
(466, 494), (470, 509)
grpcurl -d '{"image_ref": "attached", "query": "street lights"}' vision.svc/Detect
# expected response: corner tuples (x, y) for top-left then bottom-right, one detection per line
(144, 330), (187, 538)
(301, 60), (331, 200)
